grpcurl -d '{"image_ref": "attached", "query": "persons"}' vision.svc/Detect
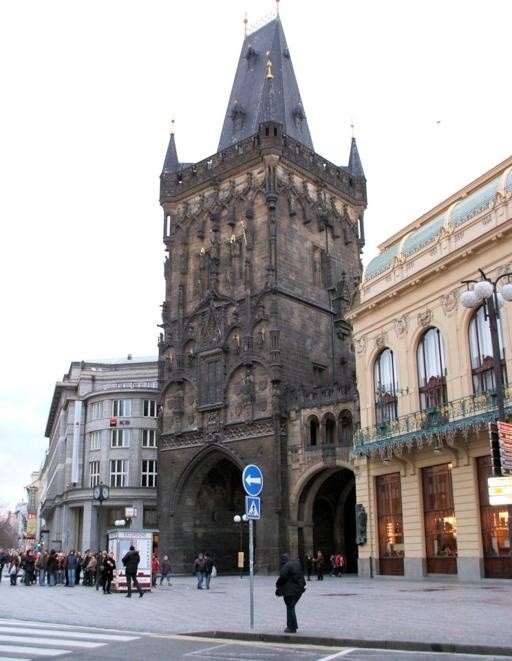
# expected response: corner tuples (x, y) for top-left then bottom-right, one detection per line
(152, 553), (160, 585)
(276, 554), (306, 633)
(122, 545), (144, 597)
(204, 553), (213, 591)
(193, 553), (205, 590)
(160, 554), (173, 587)
(0, 549), (115, 595)
(306, 549), (343, 581)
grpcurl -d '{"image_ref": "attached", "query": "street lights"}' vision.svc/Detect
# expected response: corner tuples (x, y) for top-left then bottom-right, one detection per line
(234, 513), (248, 578)
(462, 269), (512, 424)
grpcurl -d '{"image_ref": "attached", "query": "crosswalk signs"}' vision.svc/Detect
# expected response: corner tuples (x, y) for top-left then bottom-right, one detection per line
(246, 496), (262, 520)
